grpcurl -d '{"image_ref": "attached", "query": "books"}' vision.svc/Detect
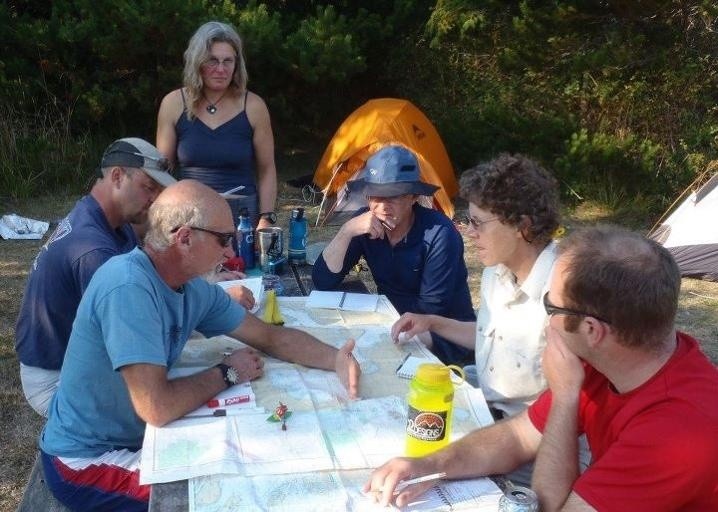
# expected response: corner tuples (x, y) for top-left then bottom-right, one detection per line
(305, 290), (379, 312)
(395, 353), (437, 381)
(216, 276), (264, 315)
(381, 478), (455, 512)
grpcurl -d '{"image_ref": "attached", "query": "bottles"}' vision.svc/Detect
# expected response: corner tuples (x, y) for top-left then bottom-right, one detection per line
(287, 207), (308, 266)
(406, 364), (465, 459)
(236, 207), (256, 270)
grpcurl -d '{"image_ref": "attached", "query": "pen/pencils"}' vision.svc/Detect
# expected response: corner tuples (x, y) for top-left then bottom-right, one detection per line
(393, 472), (447, 493)
(395, 352), (412, 373)
(380, 219), (393, 232)
(221, 266), (230, 273)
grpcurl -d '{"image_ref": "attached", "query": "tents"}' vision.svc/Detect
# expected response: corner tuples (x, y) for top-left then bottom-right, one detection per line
(311, 97), (460, 219)
(647, 159), (718, 282)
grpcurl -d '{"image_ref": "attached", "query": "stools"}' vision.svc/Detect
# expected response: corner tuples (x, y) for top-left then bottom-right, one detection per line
(17, 459), (71, 511)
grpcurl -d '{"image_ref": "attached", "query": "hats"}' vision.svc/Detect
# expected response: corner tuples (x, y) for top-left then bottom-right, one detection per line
(99, 137), (179, 190)
(344, 144), (442, 199)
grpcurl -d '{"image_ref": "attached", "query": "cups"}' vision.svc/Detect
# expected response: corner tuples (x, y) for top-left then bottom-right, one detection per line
(256, 226), (284, 273)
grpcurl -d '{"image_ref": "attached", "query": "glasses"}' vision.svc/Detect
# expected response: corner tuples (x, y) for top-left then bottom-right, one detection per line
(108, 148), (177, 177)
(463, 211), (508, 230)
(170, 224), (235, 248)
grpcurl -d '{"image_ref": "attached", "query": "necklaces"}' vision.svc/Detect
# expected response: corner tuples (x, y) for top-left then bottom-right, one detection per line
(201, 91), (225, 114)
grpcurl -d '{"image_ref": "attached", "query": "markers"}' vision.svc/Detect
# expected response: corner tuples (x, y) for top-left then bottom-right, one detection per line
(207, 394), (256, 407)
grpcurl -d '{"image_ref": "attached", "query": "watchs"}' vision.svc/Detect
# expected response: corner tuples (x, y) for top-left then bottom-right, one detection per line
(258, 212), (277, 224)
(214, 363), (240, 388)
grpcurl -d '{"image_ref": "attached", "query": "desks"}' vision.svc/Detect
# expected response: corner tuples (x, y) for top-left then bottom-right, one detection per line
(146, 265), (514, 511)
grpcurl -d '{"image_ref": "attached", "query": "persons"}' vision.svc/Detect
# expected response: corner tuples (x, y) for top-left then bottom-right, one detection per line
(391, 150), (592, 491)
(313, 144), (477, 366)
(14, 136), (256, 419)
(40, 180), (360, 512)
(361, 221), (718, 512)
(155, 21), (277, 250)
